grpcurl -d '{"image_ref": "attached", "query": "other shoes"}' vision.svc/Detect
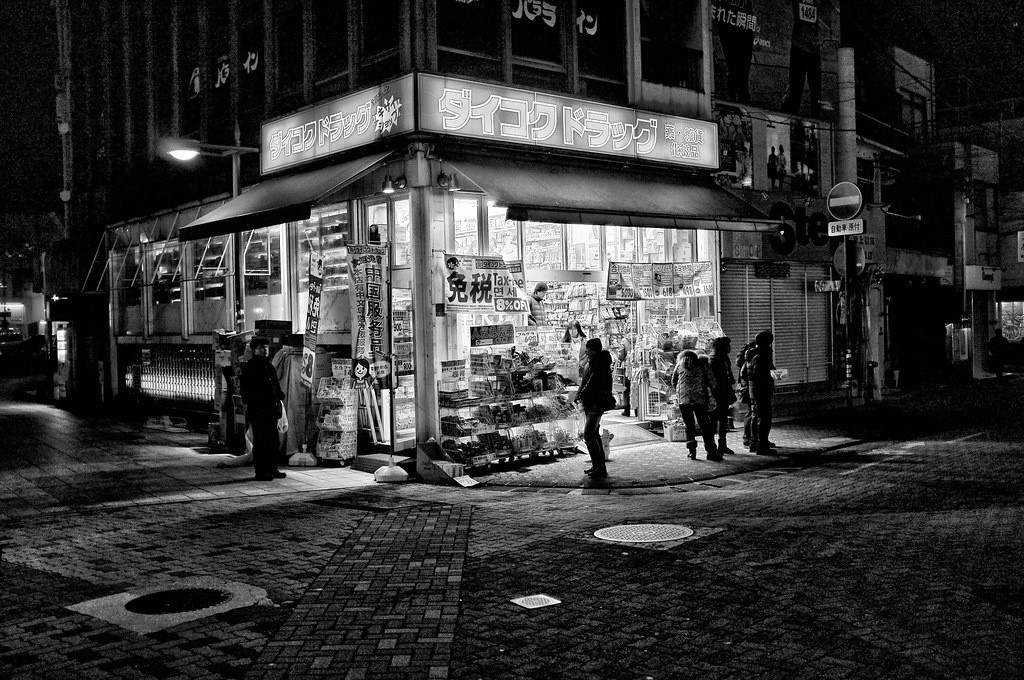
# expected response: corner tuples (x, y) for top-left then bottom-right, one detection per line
(272, 465), (287, 478)
(255, 470), (274, 481)
(588, 466), (607, 476)
(750, 443), (757, 452)
(744, 440), (749, 446)
(768, 441), (775, 448)
(756, 445), (774, 455)
(584, 462), (597, 474)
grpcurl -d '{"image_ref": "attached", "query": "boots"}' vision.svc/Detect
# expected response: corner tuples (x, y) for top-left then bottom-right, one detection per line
(687, 441), (697, 460)
(718, 439), (734, 454)
(704, 441), (722, 460)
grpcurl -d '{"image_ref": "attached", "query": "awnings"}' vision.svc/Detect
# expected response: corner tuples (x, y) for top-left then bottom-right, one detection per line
(440, 156), (784, 232)
(178, 149), (394, 243)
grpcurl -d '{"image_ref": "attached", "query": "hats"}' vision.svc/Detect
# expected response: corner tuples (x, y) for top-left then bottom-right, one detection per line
(250, 338), (270, 349)
(586, 338), (602, 352)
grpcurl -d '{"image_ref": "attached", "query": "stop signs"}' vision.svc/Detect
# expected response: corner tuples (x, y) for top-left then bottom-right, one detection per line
(827, 182), (864, 222)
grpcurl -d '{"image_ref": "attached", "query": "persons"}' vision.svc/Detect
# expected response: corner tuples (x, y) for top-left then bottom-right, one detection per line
(527, 282), (548, 326)
(617, 332), (638, 417)
(240, 337), (286, 481)
(573, 338), (613, 478)
(768, 145), (787, 192)
(988, 328), (1010, 379)
(559, 319), (590, 377)
(670, 329), (788, 462)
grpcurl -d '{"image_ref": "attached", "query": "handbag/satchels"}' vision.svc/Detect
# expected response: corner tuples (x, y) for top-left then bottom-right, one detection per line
(599, 396), (616, 411)
